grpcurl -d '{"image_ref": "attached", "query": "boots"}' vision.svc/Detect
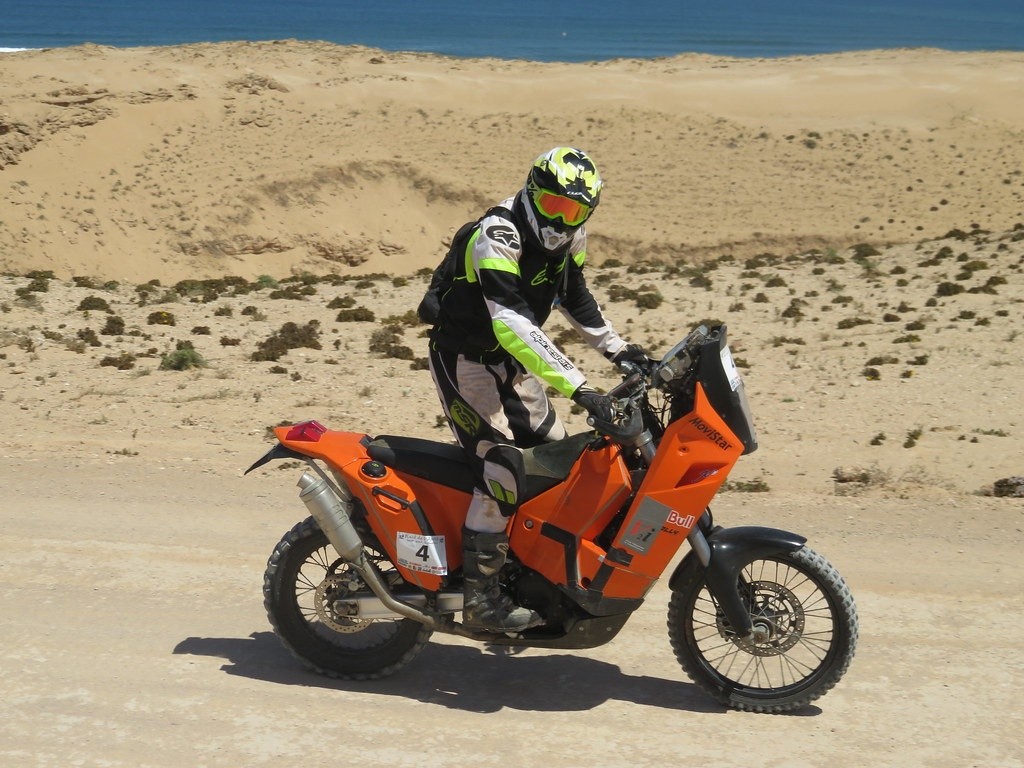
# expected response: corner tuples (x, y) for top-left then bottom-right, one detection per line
(460, 523), (542, 632)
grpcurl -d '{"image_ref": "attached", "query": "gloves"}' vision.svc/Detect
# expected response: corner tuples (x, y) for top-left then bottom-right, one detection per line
(570, 381), (620, 439)
(613, 343), (655, 372)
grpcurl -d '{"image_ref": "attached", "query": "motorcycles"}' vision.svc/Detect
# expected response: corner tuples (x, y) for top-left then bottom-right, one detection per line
(242, 324), (860, 715)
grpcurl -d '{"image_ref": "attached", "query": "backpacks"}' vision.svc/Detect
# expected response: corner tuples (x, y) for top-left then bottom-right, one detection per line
(416, 205), (528, 324)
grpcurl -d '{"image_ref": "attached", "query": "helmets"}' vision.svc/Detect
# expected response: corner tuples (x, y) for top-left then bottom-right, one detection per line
(516, 147), (602, 253)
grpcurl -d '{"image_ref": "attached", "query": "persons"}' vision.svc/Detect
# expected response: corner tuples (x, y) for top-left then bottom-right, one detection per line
(416, 147), (655, 632)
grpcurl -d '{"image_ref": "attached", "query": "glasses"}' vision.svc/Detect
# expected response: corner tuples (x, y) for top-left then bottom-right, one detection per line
(525, 166), (595, 227)
